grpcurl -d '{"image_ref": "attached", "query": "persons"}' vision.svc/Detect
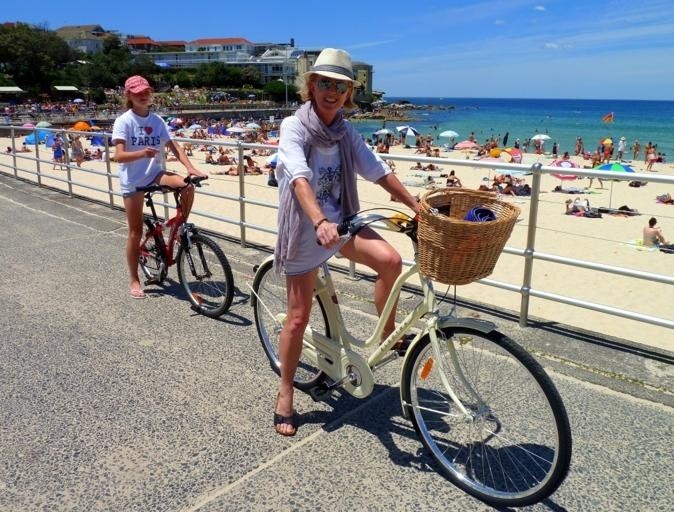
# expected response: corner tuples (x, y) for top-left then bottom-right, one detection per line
(415, 125), (439, 157)
(449, 143), (458, 150)
(534, 141), (544, 154)
(206, 145), (261, 176)
(493, 174), (529, 196)
(0, 99), (96, 116)
(468, 132), (477, 141)
(427, 176), (442, 186)
(575, 136), (584, 156)
(643, 218), (671, 246)
(515, 137), (529, 153)
(616, 137), (624, 160)
(566, 198), (592, 214)
(416, 162), (443, 172)
(113, 75), (206, 299)
(633, 140), (640, 160)
(44, 134), (102, 170)
(384, 159), (396, 172)
(275, 48), (417, 436)
(645, 142), (668, 171)
(238, 131), (268, 143)
(224, 114), (263, 125)
(551, 142), (559, 159)
(592, 137), (614, 162)
(447, 171), (463, 188)
(588, 176), (603, 189)
(562, 152), (570, 160)
(7, 142), (32, 153)
(361, 133), (402, 153)
(208, 116), (223, 140)
(391, 194), (420, 203)
(154, 95), (182, 114)
(555, 185), (588, 194)
(180, 143), (205, 157)
(478, 134), (501, 155)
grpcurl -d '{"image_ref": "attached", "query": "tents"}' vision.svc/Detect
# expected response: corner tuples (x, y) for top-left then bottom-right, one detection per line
(487, 146), (522, 162)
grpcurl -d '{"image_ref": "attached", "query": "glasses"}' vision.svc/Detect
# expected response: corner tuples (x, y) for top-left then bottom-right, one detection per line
(317, 78), (349, 95)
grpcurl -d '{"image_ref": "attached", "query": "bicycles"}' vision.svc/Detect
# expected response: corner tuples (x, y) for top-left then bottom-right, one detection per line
(244, 208), (573, 507)
(135, 175), (234, 318)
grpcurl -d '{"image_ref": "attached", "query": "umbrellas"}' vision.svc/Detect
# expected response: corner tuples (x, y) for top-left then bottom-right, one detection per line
(532, 134), (552, 140)
(23, 124), (35, 129)
(37, 120), (51, 129)
(594, 161), (634, 208)
(167, 117), (182, 129)
(265, 153), (277, 166)
(375, 129), (395, 135)
(176, 128), (185, 134)
(91, 125), (100, 131)
(75, 121), (90, 130)
(549, 159), (580, 189)
(395, 125), (420, 145)
(247, 123), (261, 129)
(189, 124), (202, 131)
(226, 127), (245, 132)
(440, 130), (459, 142)
(454, 140), (478, 159)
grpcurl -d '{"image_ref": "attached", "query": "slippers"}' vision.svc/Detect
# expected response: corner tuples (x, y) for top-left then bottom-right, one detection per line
(273, 391), (297, 438)
(127, 282), (147, 300)
(378, 333), (417, 357)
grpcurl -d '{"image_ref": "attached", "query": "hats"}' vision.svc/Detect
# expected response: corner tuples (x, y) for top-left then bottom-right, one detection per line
(302, 47), (362, 87)
(123, 75), (154, 94)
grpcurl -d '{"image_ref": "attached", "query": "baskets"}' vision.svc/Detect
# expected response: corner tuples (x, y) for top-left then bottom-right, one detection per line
(416, 186), (522, 286)
